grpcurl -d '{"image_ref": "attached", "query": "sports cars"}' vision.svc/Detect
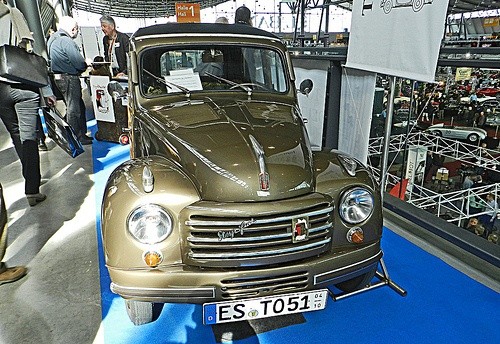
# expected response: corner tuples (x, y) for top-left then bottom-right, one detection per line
(427, 122), (488, 143)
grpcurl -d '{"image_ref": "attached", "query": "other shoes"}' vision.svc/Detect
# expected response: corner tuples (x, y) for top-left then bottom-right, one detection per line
(37, 138), (48, 151)
(0, 266), (26, 285)
(79, 134), (94, 144)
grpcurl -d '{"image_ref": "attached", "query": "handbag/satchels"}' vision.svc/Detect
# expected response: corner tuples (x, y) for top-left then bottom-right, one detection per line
(44, 99), (85, 158)
(0, 22), (48, 88)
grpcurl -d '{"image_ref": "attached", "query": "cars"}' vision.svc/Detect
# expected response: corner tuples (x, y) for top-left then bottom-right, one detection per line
(101, 22), (384, 327)
(460, 86), (500, 125)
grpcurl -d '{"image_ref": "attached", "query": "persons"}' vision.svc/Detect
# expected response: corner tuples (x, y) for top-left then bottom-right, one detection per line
(421, 98), (430, 122)
(428, 103), (436, 124)
(400, 61), (500, 110)
(438, 102), (445, 118)
(411, 98), (419, 115)
(0, 1), (49, 207)
(485, 193), (499, 222)
(99, 15), (131, 81)
(382, 106), (387, 119)
(462, 174), (488, 206)
(479, 111), (486, 130)
(488, 234), (498, 244)
(0, 182), (27, 285)
(468, 218), (478, 225)
(431, 146), (446, 183)
(46, 15), (92, 146)
(383, 93), (389, 105)
(468, 224), (489, 239)
(223, 6), (255, 81)
(423, 149), (433, 180)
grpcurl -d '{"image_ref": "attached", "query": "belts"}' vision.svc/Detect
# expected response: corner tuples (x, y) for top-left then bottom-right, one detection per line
(53, 72), (81, 81)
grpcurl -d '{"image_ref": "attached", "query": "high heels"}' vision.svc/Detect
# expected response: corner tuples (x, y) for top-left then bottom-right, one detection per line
(28, 195), (46, 207)
